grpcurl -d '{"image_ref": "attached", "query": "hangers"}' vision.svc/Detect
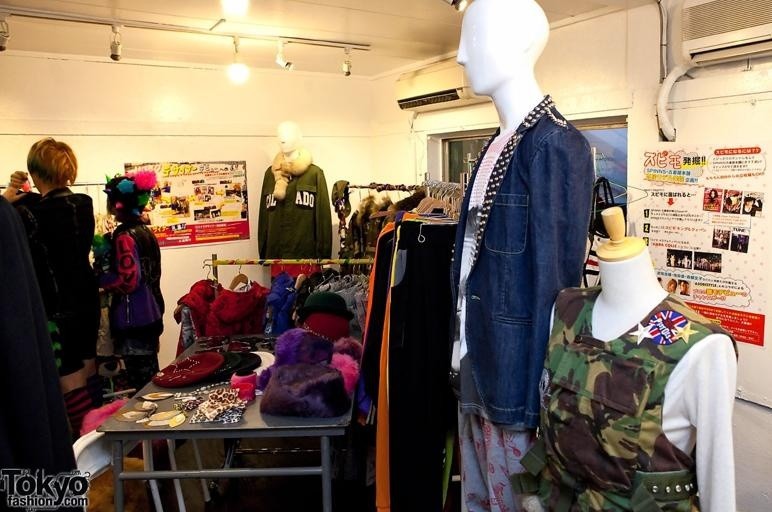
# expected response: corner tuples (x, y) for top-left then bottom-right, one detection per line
(415, 179), (465, 222)
(590, 153), (649, 212)
(202, 258), (370, 291)
(594, 177), (627, 203)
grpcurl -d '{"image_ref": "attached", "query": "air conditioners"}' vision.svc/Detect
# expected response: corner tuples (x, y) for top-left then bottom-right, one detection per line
(391, 61), (491, 112)
(676, 0), (772, 71)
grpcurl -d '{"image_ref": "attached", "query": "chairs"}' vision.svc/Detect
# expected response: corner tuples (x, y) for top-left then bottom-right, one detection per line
(72, 419), (165, 512)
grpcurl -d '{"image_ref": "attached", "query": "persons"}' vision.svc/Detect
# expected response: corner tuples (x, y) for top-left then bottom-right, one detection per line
(3, 135), (104, 443)
(93, 169), (167, 392)
(725, 190), (742, 212)
(666, 279), (677, 294)
(706, 190), (719, 210)
(445, 0), (597, 512)
(511, 205), (739, 512)
(679, 281), (690, 296)
(255, 122), (334, 257)
(743, 196), (762, 217)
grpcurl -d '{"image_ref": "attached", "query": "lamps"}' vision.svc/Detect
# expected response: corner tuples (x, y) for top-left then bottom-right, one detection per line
(0, 13), (11, 52)
(275, 39), (293, 70)
(109, 24), (122, 62)
(343, 49), (352, 77)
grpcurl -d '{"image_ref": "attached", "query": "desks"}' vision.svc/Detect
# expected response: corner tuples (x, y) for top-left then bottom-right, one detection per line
(96, 322), (354, 512)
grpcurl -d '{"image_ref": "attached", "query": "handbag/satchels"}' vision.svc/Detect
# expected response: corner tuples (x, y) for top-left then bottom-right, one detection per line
(589, 200), (626, 238)
(109, 285), (163, 329)
(581, 231), (610, 290)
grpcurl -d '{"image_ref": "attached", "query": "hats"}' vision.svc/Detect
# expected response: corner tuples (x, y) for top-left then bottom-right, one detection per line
(333, 336), (363, 362)
(329, 352), (360, 394)
(106, 170), (157, 219)
(296, 293), (354, 319)
(260, 362), (351, 417)
(303, 314), (349, 344)
(332, 180), (351, 217)
(257, 329), (334, 389)
